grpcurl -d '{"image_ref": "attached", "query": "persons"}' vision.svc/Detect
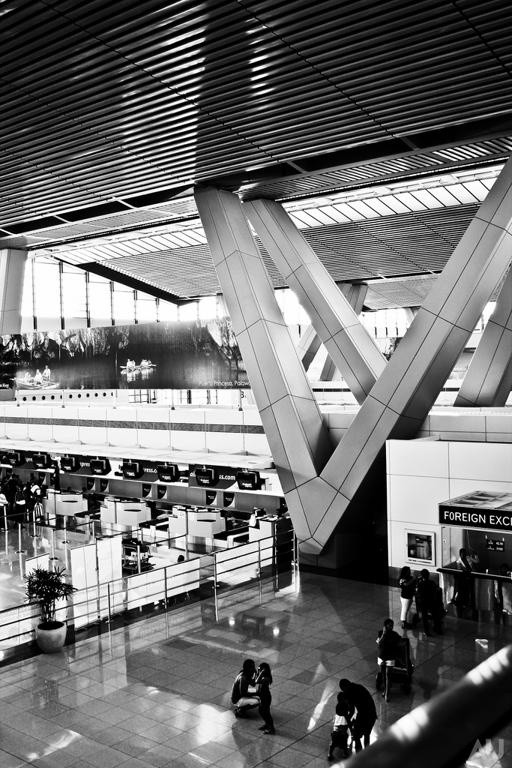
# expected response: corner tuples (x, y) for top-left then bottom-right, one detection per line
(24, 365), (51, 385)
(126, 358), (153, 366)
(396, 567), (438, 629)
(328, 679), (377, 760)
(232, 659), (275, 734)
(0, 471), (46, 529)
(458, 548), (479, 571)
(377, 619), (404, 702)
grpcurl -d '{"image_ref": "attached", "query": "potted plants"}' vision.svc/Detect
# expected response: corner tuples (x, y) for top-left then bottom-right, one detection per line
(21, 564), (79, 653)
